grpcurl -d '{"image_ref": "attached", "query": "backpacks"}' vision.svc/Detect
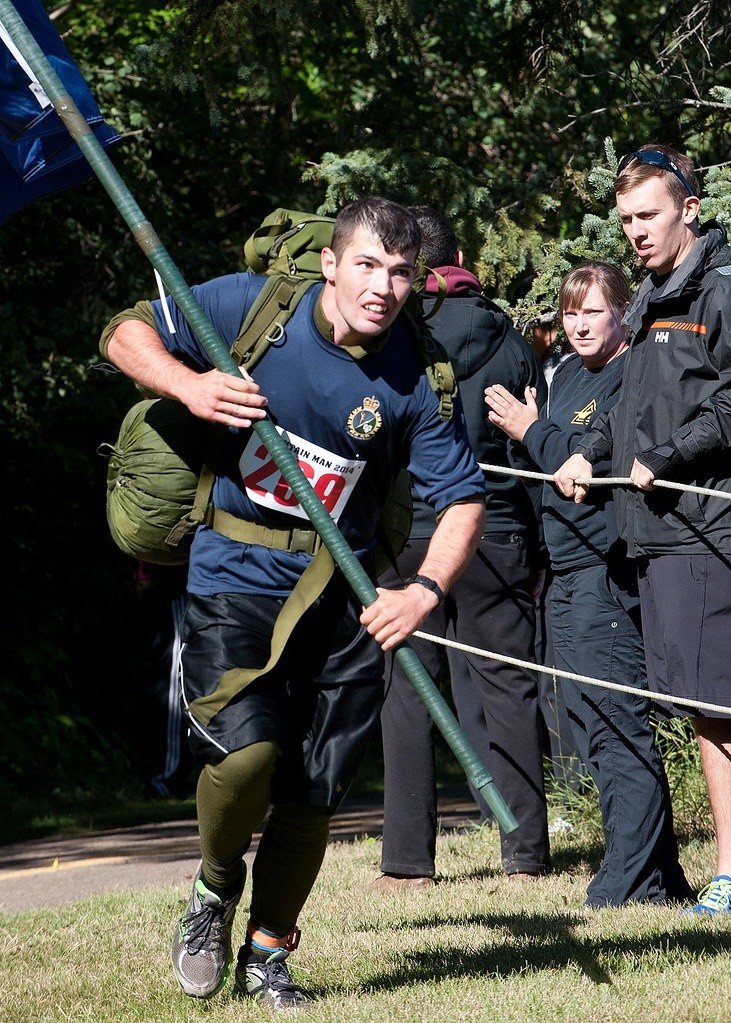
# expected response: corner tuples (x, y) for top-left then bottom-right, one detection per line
(97, 207), (459, 563)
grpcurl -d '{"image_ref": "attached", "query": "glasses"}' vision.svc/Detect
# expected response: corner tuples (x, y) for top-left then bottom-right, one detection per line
(616, 148), (694, 197)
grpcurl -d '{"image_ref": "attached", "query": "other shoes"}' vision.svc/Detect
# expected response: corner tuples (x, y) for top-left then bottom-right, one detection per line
(512, 872), (535, 885)
(352, 871), (433, 898)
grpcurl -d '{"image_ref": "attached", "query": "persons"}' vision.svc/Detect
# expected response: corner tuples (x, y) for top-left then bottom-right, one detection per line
(352, 207), (547, 894)
(97, 199), (487, 1004)
(486, 141), (731, 918)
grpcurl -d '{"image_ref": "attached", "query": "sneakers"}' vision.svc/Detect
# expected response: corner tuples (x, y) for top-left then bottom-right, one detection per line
(677, 874), (731, 918)
(172, 859), (249, 1000)
(232, 944), (308, 1014)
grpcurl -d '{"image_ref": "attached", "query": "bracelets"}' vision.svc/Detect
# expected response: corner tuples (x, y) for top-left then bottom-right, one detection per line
(405, 573), (444, 610)
